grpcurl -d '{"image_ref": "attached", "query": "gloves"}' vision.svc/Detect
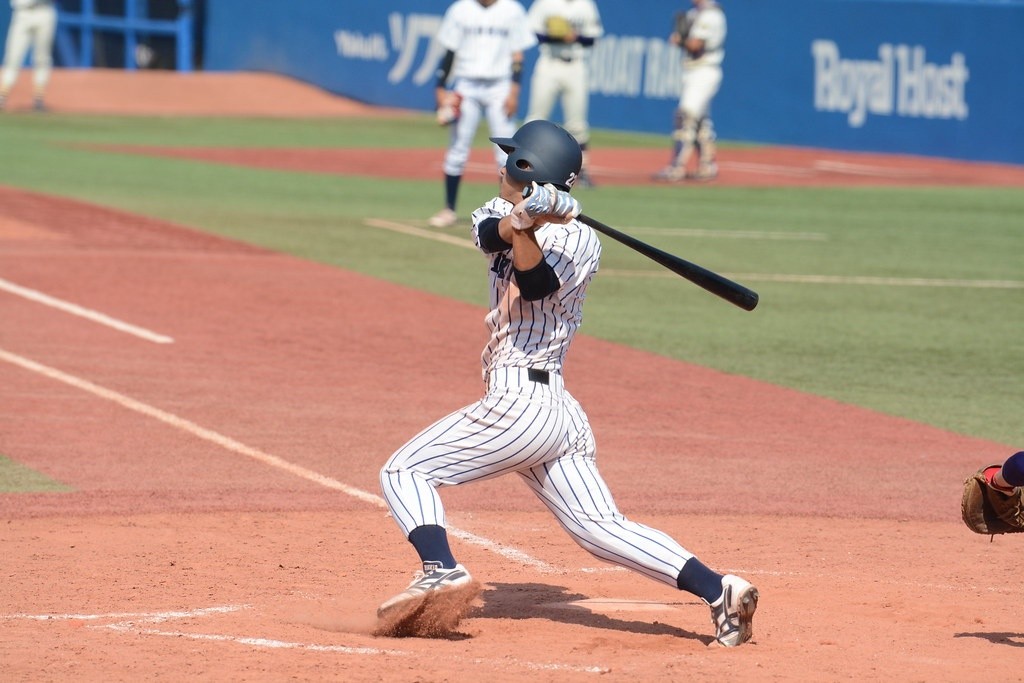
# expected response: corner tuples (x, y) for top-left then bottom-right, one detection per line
(510, 181), (582, 230)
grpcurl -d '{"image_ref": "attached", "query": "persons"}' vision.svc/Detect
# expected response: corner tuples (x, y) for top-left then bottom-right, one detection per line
(376, 121), (762, 648)
(655, 0), (728, 183)
(0, 0), (57, 114)
(429, 0), (539, 229)
(523, 0), (605, 189)
(961, 451), (1024, 535)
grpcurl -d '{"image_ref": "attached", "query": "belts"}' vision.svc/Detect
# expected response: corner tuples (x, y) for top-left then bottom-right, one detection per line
(528, 368), (549, 385)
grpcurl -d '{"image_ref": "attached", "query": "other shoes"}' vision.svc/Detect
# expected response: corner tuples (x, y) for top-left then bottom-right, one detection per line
(652, 163), (686, 182)
(428, 209), (457, 227)
(576, 170), (589, 191)
(689, 163), (717, 179)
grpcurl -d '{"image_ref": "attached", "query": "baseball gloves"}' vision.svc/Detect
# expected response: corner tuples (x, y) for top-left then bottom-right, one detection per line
(961, 464), (1024, 543)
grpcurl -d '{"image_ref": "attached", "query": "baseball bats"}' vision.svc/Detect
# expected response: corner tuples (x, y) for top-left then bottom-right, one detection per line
(521, 185), (759, 313)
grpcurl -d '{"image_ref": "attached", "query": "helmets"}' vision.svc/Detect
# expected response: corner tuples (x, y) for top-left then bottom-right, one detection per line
(489, 119), (582, 193)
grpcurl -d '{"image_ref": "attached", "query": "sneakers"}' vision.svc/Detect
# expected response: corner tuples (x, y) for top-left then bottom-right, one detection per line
(700, 573), (757, 648)
(377, 561), (472, 619)
